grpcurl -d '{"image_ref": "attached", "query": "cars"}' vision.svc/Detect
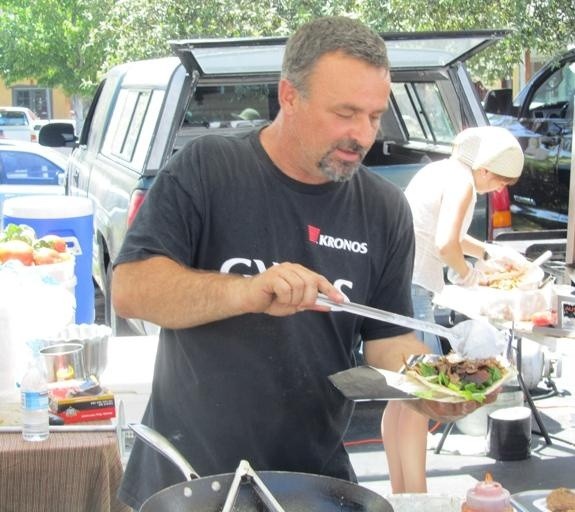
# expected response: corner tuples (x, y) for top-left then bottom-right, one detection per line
(0, 139), (70, 218)
(0, 107), (40, 143)
(485, 49), (575, 221)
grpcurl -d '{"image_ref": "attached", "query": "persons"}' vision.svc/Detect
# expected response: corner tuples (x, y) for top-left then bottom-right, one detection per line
(380, 123), (525, 495)
(110, 15), (501, 512)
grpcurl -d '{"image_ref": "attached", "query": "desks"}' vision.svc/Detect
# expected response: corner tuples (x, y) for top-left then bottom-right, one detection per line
(0, 430), (130, 512)
(432, 286), (574, 460)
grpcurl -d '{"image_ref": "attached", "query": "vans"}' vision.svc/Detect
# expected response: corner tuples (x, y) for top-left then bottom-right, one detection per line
(39, 29), (507, 336)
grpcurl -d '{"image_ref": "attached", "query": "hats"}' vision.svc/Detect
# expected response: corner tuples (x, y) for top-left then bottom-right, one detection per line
(450, 126), (525, 179)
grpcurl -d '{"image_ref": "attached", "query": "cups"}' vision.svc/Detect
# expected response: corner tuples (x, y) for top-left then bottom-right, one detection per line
(41, 344), (84, 378)
(484, 414), (532, 462)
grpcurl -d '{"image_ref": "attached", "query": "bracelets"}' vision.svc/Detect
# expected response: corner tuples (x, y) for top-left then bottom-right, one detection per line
(483, 250), (488, 260)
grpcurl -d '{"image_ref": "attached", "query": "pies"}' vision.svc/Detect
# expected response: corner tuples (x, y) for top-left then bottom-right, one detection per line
(405, 357), (513, 399)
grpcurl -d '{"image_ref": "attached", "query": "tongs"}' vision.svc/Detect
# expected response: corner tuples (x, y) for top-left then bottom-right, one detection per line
(218, 460), (286, 511)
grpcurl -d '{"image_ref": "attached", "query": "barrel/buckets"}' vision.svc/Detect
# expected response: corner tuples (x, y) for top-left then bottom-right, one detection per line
(2, 194), (95, 325)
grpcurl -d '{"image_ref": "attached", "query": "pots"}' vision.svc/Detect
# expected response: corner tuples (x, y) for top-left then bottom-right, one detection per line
(127, 418), (393, 512)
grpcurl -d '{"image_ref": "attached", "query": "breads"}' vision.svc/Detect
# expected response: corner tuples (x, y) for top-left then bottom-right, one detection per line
(546, 488), (575, 511)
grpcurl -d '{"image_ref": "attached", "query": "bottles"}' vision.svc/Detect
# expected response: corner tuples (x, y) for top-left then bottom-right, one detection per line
(459, 473), (514, 512)
(20, 359), (50, 443)
